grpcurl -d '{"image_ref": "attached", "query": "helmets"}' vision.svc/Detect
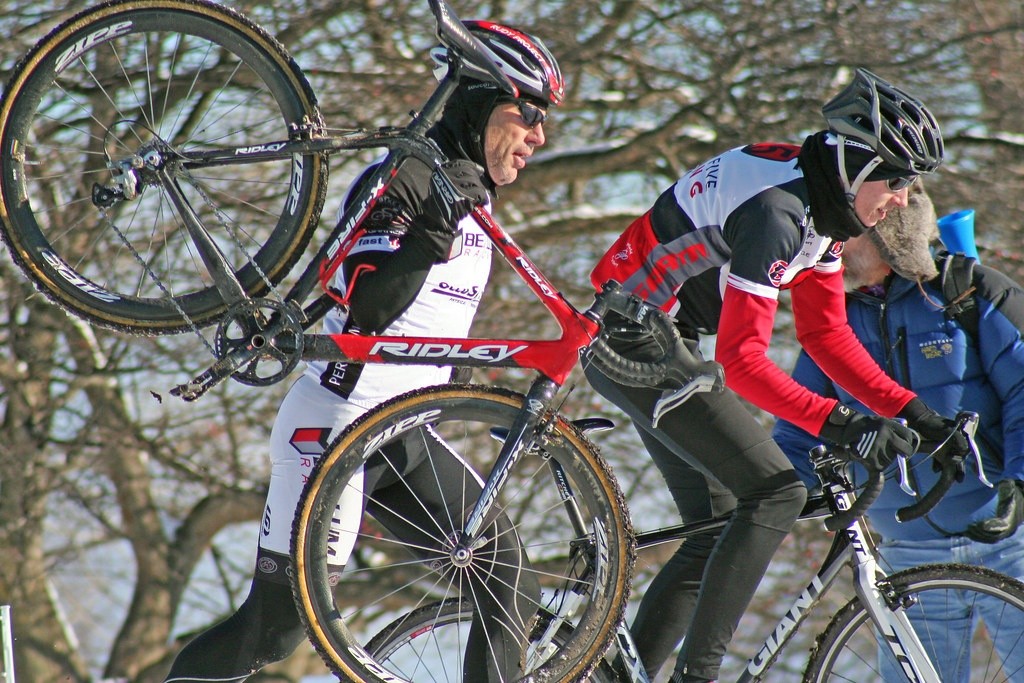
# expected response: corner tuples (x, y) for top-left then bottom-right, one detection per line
(428, 18), (565, 110)
(820, 66), (945, 176)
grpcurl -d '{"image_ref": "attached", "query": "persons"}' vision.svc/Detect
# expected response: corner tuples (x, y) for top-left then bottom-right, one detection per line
(770, 174), (1024, 683)
(159, 20), (568, 683)
(581, 66), (965, 683)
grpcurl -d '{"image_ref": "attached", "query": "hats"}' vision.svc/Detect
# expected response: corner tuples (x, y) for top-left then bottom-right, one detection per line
(867, 177), (944, 310)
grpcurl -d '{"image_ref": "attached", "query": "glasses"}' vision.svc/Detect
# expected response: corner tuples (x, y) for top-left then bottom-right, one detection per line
(504, 98), (550, 130)
(886, 176), (920, 193)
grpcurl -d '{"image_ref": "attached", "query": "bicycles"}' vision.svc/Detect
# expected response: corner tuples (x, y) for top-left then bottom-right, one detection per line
(358, 410), (1024, 683)
(0, 1), (726, 683)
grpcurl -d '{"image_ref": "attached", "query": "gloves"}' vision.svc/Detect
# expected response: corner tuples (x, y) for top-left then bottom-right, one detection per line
(964, 478), (1024, 545)
(894, 396), (971, 483)
(818, 400), (913, 473)
(399, 159), (490, 265)
